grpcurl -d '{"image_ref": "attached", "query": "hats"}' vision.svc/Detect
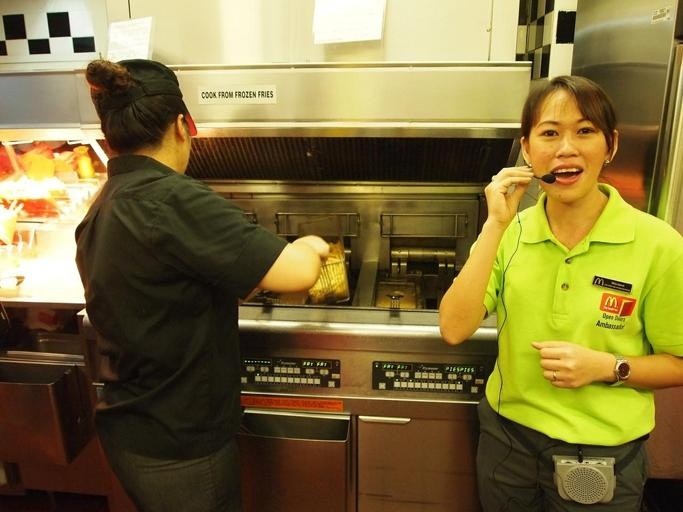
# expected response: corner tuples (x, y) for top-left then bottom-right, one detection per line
(90, 59), (198, 138)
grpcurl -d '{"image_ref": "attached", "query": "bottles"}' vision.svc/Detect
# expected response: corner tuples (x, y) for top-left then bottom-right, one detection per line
(72, 144), (94, 179)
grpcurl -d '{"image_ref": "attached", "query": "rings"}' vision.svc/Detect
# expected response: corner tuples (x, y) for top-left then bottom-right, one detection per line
(551, 370), (560, 382)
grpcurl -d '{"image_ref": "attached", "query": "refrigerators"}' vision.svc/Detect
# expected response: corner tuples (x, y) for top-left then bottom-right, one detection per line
(572, 0), (683, 480)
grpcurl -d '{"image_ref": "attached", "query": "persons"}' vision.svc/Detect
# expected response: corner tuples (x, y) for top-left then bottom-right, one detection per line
(437, 75), (682, 511)
(73, 58), (331, 512)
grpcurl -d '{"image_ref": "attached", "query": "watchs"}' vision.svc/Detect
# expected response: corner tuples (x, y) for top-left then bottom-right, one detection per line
(608, 354), (631, 390)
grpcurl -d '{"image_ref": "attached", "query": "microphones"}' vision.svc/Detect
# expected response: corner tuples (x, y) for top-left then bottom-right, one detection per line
(522, 160), (556, 186)
